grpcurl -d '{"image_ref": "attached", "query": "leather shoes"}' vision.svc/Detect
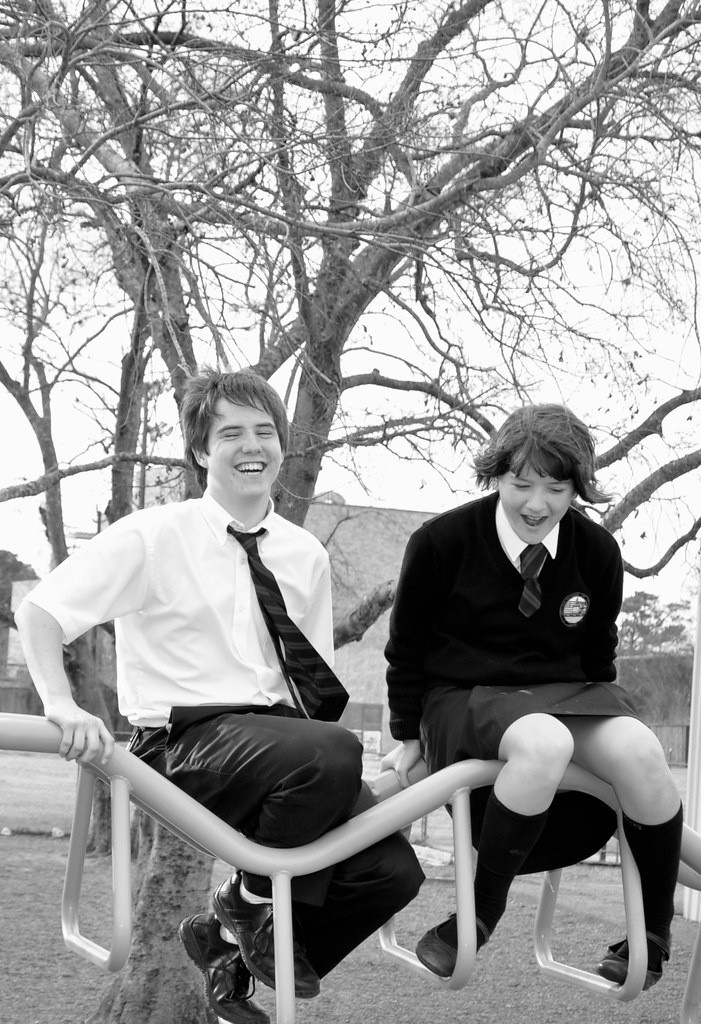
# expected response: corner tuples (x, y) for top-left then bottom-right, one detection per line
(211, 869), (321, 998)
(180, 914), (271, 1024)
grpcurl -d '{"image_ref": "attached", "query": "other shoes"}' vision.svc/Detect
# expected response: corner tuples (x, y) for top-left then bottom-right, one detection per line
(416, 913), (490, 977)
(597, 931), (671, 990)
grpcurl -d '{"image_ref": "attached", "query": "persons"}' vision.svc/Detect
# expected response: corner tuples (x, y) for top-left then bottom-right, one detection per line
(379, 403), (683, 992)
(14, 369), (427, 1024)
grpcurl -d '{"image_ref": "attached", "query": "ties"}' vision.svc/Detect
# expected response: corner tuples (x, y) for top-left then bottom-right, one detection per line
(518, 542), (548, 617)
(227, 525), (350, 722)
